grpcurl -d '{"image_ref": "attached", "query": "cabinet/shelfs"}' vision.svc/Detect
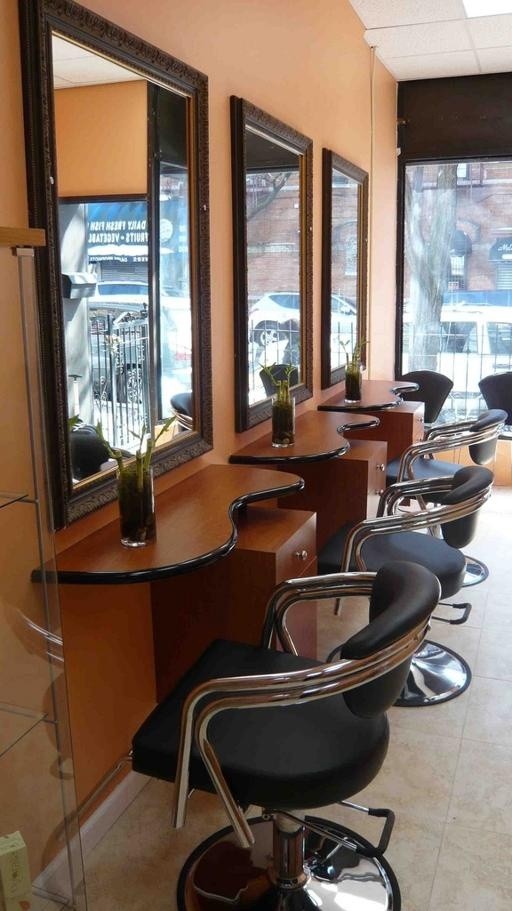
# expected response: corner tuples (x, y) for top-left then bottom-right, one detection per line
(3, 227), (92, 911)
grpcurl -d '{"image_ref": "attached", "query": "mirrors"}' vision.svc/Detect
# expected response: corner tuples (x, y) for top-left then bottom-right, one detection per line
(321, 148), (368, 393)
(230, 95), (313, 437)
(19, 0), (214, 535)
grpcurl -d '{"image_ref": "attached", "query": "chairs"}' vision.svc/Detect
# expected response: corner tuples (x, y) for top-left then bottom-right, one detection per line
(478, 372), (511, 439)
(399, 369), (454, 432)
(132, 560), (443, 910)
(315, 466), (494, 706)
(385, 409), (508, 585)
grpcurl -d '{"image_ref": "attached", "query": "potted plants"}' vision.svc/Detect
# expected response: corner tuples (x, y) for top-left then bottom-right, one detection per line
(258, 359), (301, 449)
(83, 414), (178, 547)
(339, 333), (371, 403)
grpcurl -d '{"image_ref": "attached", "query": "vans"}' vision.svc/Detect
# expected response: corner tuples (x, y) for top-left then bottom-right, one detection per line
(400, 306), (512, 394)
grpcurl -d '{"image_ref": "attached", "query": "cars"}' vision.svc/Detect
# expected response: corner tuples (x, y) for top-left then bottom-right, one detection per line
(84, 300), (174, 411)
(159, 297), (192, 372)
(248, 291), (359, 348)
(86, 279), (174, 306)
